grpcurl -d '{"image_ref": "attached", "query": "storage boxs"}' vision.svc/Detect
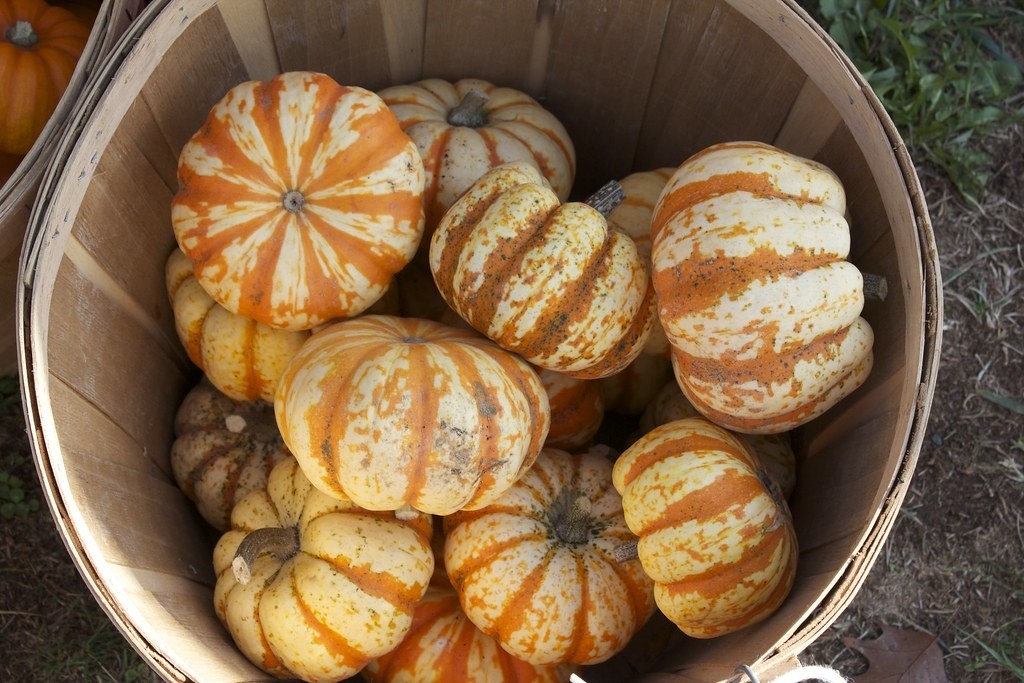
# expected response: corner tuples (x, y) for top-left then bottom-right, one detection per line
(0, 0), (946, 682)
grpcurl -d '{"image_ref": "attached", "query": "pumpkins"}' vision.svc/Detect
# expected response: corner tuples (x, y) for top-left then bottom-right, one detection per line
(0, 0), (883, 683)
